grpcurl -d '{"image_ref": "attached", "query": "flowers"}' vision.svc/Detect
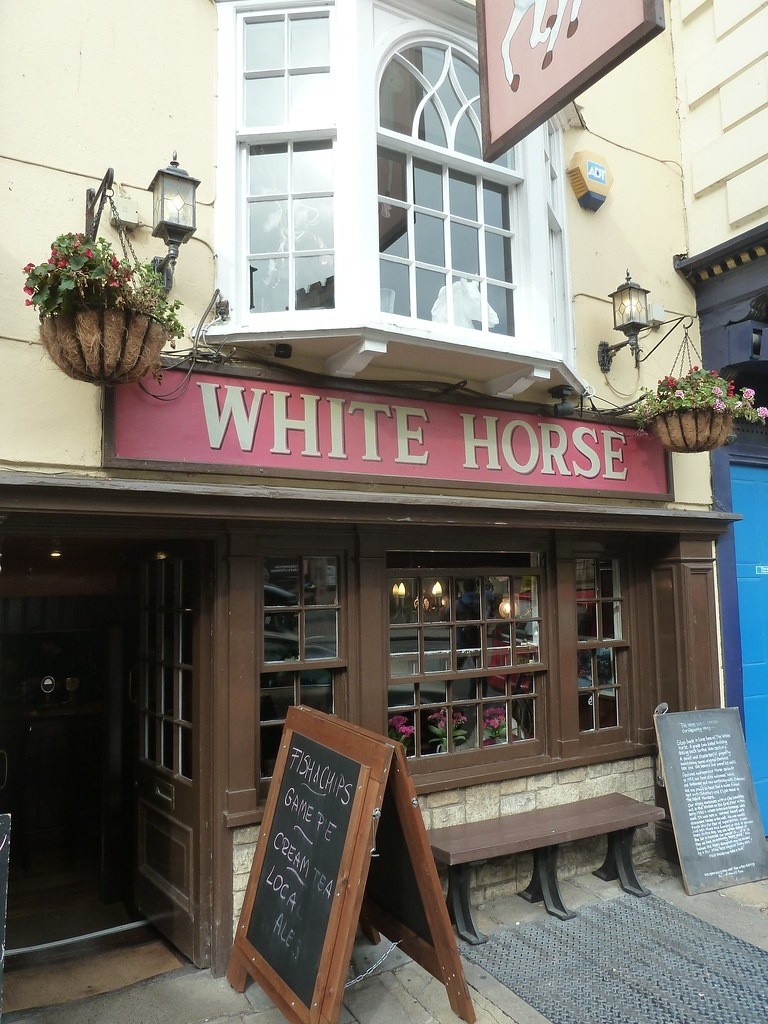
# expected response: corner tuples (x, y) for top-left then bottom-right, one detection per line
(634, 366), (768, 439)
(388, 715), (414, 746)
(23, 233), (184, 340)
(428, 710), (467, 750)
(482, 708), (506, 739)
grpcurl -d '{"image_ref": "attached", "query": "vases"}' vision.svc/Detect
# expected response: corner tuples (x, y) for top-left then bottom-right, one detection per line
(39, 302), (168, 386)
(437, 743), (455, 753)
(649, 407), (733, 453)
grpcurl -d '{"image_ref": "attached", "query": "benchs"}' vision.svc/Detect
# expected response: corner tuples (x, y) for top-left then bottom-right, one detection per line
(427, 793), (665, 944)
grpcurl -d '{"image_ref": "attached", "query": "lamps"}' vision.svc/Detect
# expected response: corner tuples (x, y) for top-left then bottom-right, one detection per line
(500, 577), (517, 618)
(598, 268), (650, 372)
(392, 580), (445, 620)
(83, 151), (201, 294)
